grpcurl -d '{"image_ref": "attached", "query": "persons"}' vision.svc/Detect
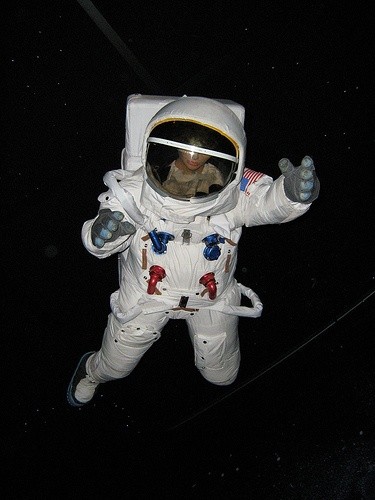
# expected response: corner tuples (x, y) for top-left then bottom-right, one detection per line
(67, 91), (322, 413)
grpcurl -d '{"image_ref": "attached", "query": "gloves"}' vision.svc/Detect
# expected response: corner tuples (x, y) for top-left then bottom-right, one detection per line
(278, 155), (320, 204)
(91, 208), (137, 249)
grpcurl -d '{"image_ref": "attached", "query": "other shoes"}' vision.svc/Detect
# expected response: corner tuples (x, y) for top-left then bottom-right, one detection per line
(68, 350), (100, 407)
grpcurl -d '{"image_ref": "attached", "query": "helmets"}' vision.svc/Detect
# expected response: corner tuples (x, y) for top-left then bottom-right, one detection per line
(141, 96), (247, 221)
